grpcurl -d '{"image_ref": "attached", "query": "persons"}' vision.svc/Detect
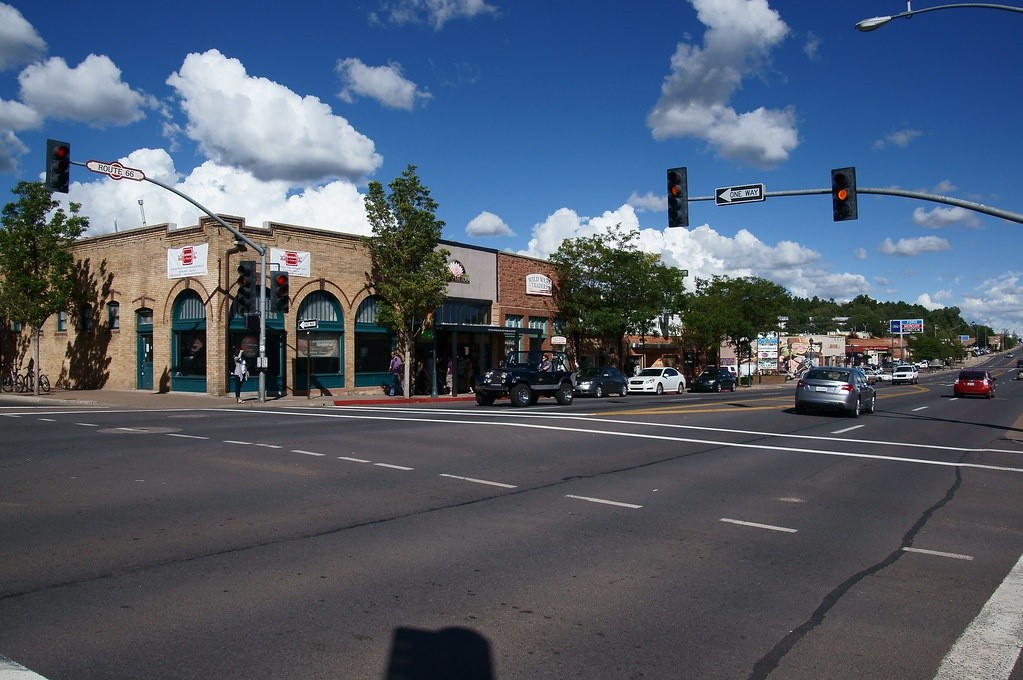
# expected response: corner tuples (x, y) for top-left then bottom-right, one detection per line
(879, 357), (955, 376)
(233, 349), (248, 404)
(389, 351), (551, 396)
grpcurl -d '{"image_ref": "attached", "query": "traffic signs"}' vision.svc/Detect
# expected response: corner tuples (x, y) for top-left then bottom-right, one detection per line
(714, 183), (764, 206)
(298, 319), (318, 330)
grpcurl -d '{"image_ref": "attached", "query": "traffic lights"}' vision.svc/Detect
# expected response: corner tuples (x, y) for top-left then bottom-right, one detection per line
(271, 271), (289, 313)
(666, 166), (689, 228)
(46, 138), (70, 194)
(236, 260), (257, 314)
(830, 167), (858, 222)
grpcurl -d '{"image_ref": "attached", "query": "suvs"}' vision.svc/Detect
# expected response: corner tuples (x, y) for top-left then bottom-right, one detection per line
(473, 349), (578, 408)
(892, 365), (919, 385)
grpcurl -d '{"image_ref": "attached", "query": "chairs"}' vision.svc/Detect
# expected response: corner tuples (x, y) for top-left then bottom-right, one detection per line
(837, 375), (848, 382)
(816, 373), (829, 379)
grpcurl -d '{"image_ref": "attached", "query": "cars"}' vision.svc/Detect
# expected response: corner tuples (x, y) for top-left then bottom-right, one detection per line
(795, 366), (877, 418)
(770, 369), (795, 380)
(863, 366), (885, 383)
(690, 369), (738, 393)
(1017, 372), (1023, 380)
(1016, 360), (1023, 368)
(627, 366), (687, 395)
(954, 369), (997, 399)
(571, 367), (629, 398)
(1007, 352), (1015, 358)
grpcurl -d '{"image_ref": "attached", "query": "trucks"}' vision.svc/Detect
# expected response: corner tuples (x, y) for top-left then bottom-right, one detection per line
(914, 357), (945, 369)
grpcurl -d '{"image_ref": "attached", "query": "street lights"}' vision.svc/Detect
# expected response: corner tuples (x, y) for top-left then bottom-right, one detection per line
(809, 337), (813, 370)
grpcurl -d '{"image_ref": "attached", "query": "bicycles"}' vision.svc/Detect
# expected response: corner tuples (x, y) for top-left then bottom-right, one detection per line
(23, 366), (50, 392)
(1, 363), (25, 393)
